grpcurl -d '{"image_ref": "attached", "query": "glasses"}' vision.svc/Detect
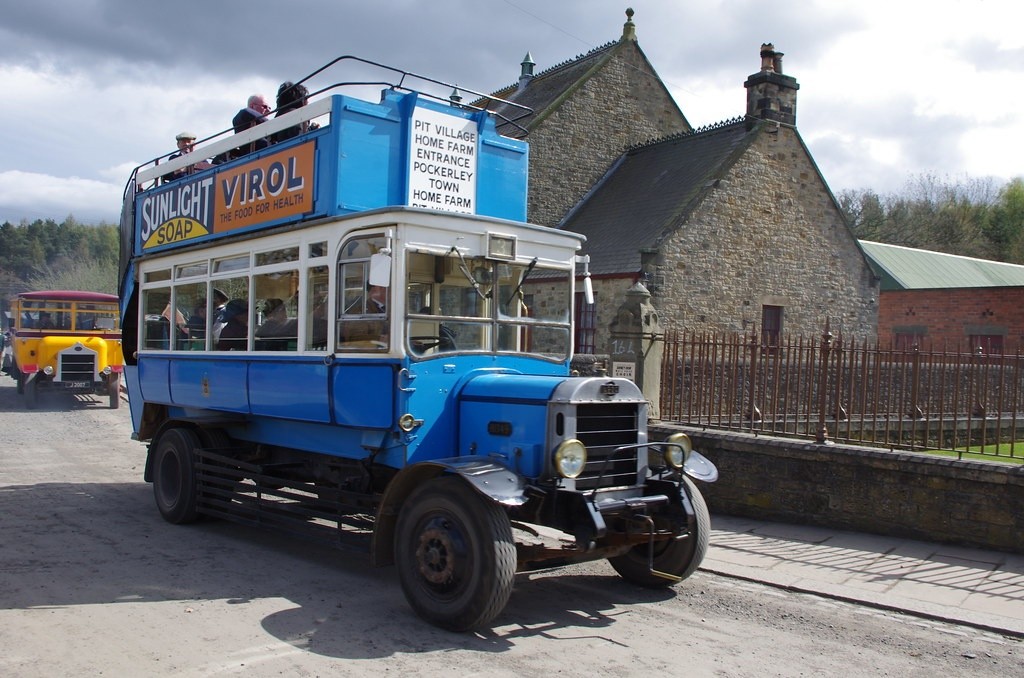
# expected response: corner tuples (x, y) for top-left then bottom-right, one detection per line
(253, 102), (267, 109)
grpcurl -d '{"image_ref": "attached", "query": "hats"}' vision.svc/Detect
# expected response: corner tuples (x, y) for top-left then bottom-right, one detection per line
(262, 299), (284, 317)
(176, 131), (195, 141)
(223, 298), (250, 322)
(212, 286), (229, 299)
(40, 312), (51, 317)
(366, 279), (375, 292)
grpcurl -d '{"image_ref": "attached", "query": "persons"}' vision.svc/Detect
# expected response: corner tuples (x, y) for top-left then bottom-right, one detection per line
(280, 289), (328, 352)
(182, 298), (214, 351)
(230, 94), (272, 154)
(217, 299), (249, 351)
(273, 83), (320, 141)
(344, 278), (393, 342)
(255, 298), (288, 351)
(34, 313), (54, 328)
(161, 133), (197, 181)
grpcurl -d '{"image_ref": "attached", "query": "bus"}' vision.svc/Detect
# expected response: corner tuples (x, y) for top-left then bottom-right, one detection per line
(11, 291), (122, 410)
(117, 56), (717, 633)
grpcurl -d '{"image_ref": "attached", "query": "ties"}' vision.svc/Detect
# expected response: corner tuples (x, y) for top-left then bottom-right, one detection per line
(381, 304), (387, 313)
